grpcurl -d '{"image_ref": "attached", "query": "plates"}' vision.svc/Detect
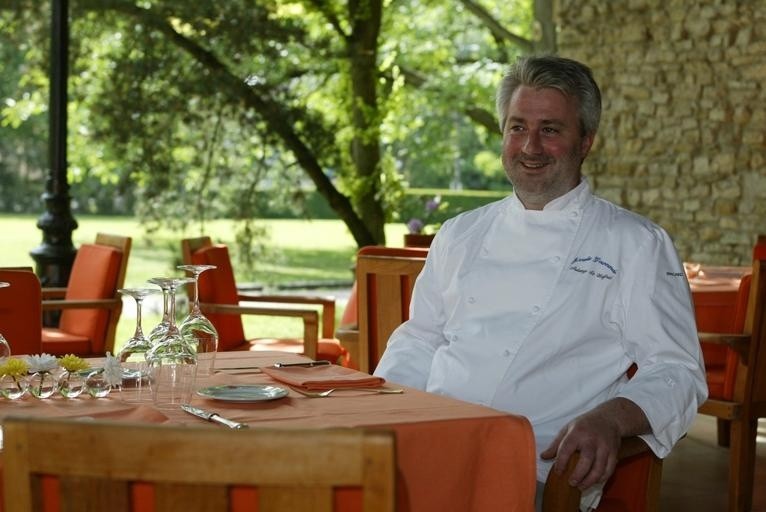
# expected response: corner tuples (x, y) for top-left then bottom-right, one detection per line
(75, 364), (147, 384)
(195, 382), (287, 402)
(689, 277), (733, 288)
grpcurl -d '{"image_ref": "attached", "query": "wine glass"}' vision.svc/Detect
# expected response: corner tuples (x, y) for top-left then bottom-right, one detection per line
(117, 286), (163, 404)
(174, 265), (220, 379)
(0, 281), (12, 371)
(148, 275), (197, 412)
(144, 277), (185, 403)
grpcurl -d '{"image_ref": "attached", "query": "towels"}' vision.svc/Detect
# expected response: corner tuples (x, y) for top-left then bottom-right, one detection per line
(262, 361), (387, 392)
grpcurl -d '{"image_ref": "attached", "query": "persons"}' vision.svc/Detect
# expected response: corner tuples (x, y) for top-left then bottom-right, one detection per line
(369, 56), (709, 511)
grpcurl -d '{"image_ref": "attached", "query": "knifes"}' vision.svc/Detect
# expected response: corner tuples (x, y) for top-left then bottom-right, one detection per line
(181, 404), (245, 431)
(210, 360), (331, 372)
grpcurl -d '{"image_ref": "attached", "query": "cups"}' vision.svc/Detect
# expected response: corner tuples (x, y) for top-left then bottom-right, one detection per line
(682, 263), (701, 281)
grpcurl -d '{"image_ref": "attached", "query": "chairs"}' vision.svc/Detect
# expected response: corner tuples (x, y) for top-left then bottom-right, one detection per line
(0, 266), (40, 354)
(540, 434), (668, 511)
(693, 260), (765, 512)
(40, 231), (132, 355)
(337, 246), (431, 375)
(182, 236), (350, 365)
(1, 415), (394, 512)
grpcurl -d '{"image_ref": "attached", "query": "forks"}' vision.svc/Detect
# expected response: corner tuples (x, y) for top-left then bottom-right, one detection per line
(286, 384), (406, 399)
